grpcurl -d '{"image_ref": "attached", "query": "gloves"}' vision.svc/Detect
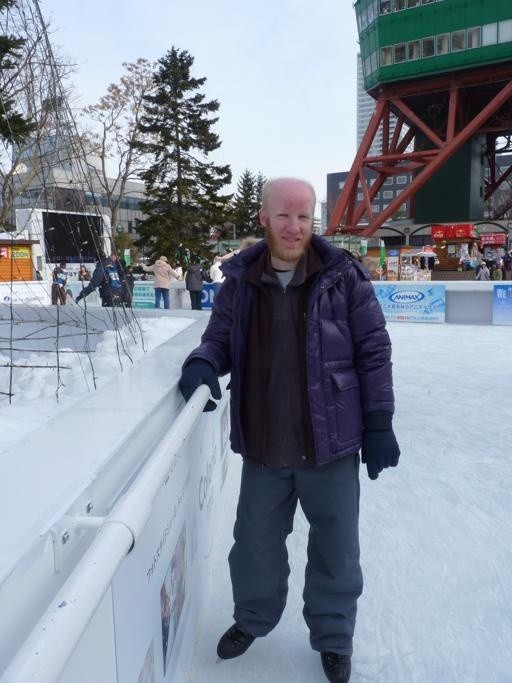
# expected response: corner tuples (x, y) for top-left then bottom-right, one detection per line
(177, 361), (222, 413)
(358, 425), (402, 481)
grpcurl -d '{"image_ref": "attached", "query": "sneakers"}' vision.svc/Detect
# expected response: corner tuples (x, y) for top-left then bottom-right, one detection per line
(320, 651), (352, 683)
(217, 624), (257, 659)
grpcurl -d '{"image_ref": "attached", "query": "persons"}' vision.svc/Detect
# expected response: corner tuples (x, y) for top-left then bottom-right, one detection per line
(380, 258), (388, 281)
(51, 251), (225, 312)
(227, 247), (235, 256)
(180, 177), (402, 682)
(462, 251), (502, 280)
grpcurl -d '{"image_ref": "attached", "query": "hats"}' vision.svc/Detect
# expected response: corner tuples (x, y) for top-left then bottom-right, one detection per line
(159, 255), (168, 262)
(481, 262), (486, 265)
